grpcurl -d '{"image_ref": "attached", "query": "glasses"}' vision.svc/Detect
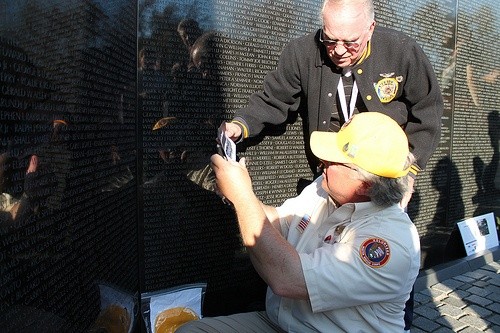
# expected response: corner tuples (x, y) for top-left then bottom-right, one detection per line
(319, 25), (369, 50)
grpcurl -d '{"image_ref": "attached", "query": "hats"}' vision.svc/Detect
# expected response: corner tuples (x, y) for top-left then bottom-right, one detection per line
(310, 111), (411, 179)
(152, 117), (185, 132)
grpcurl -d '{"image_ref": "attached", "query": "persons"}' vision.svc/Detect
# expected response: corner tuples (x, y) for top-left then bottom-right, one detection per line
(215, 0), (443, 332)
(173, 111), (422, 332)
(0, 18), (236, 264)
